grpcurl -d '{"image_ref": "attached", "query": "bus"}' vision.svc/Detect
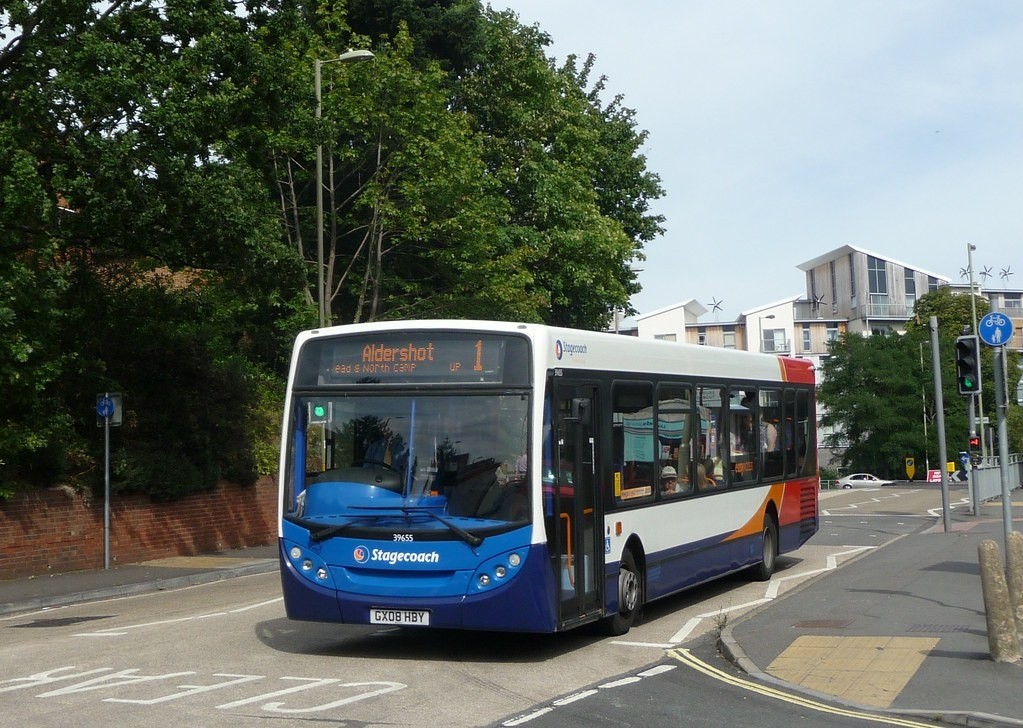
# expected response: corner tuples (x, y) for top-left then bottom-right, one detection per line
(275, 316), (820, 638)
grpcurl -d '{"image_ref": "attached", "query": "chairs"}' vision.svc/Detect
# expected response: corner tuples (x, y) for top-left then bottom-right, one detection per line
(770, 417), (801, 452)
(625, 478), (717, 498)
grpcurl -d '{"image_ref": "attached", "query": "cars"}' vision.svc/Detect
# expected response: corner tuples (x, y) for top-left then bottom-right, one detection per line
(834, 473), (896, 490)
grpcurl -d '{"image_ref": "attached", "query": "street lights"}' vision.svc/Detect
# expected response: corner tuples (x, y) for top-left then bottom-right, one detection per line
(919, 340), (930, 475)
(315, 45), (378, 472)
(966, 243), (987, 465)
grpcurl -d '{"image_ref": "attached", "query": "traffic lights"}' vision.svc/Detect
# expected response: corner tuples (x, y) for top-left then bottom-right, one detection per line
(308, 401), (332, 424)
(969, 436), (980, 453)
(955, 336), (981, 395)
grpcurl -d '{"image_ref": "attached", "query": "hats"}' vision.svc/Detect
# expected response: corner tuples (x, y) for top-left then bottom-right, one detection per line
(661, 466), (677, 478)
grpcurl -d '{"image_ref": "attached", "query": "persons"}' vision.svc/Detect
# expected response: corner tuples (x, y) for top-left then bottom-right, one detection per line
(657, 395), (777, 495)
(362, 414), (413, 472)
